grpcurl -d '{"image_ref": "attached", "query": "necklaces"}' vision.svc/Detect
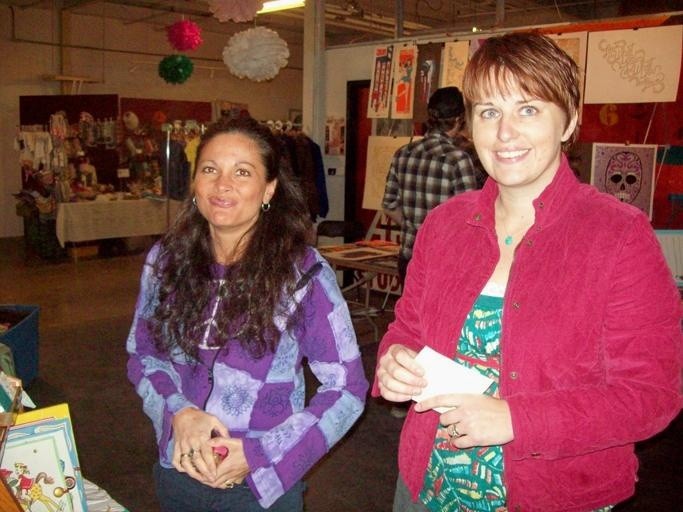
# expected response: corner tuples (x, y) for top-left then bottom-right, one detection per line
(491, 217), (535, 250)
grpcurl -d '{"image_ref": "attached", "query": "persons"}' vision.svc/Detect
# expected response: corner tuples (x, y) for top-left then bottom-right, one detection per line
(420, 114), (488, 189)
(125, 109), (369, 509)
(380, 85), (480, 423)
(369, 25), (680, 512)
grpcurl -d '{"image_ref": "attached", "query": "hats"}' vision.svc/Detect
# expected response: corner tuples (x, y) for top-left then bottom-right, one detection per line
(426, 87), (465, 119)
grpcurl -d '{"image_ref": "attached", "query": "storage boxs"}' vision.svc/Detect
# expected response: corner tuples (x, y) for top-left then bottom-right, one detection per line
(0, 303), (40, 389)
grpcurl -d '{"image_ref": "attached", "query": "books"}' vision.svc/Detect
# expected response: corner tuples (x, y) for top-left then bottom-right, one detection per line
(1, 419), (90, 512)
(2, 435), (76, 511)
(1, 393), (74, 426)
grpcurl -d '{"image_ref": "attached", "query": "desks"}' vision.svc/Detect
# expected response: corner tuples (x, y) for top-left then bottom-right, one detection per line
(318, 239), (400, 344)
(21, 193), (187, 264)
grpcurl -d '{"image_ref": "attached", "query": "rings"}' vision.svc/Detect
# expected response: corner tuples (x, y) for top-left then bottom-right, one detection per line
(447, 425), (460, 437)
(222, 480), (235, 490)
(178, 451), (193, 460)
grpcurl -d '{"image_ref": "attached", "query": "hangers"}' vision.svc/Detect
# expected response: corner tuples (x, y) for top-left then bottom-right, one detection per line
(171, 126), (198, 143)
(267, 120), (300, 134)
